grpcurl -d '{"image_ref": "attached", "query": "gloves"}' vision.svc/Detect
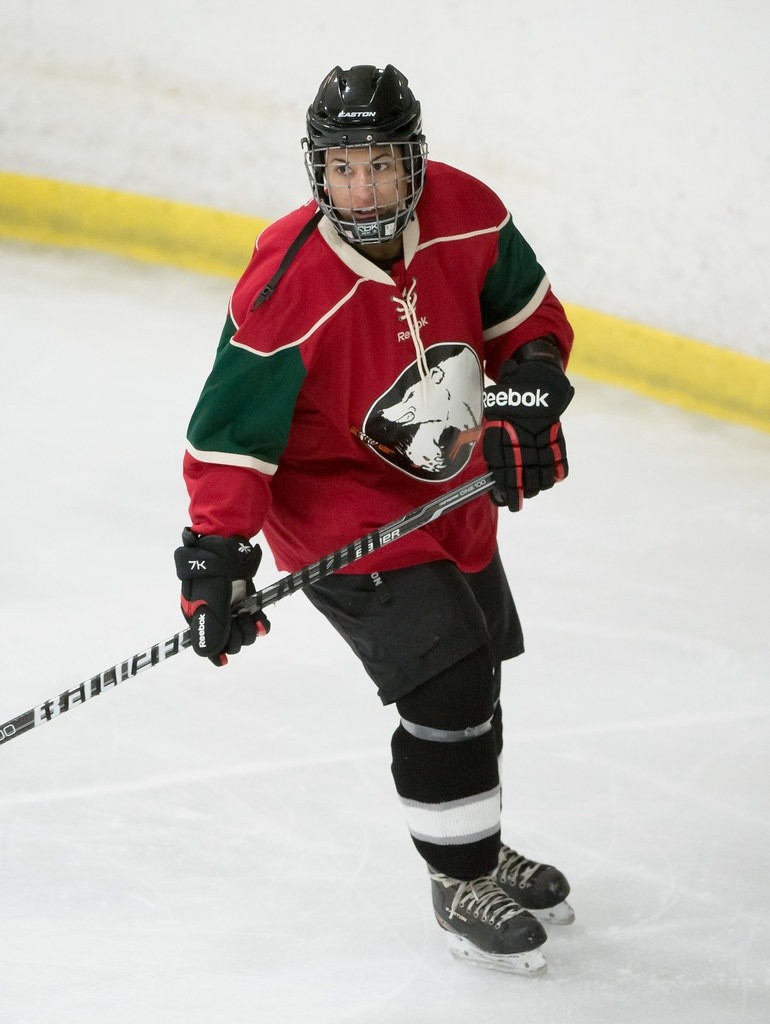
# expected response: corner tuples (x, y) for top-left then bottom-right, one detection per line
(175, 528), (274, 666)
(486, 342), (578, 504)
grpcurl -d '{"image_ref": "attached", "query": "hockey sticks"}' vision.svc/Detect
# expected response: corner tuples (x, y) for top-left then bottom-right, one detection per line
(1, 473), (499, 739)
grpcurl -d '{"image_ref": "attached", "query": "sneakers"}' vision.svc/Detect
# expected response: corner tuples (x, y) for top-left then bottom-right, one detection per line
(431, 840), (575, 979)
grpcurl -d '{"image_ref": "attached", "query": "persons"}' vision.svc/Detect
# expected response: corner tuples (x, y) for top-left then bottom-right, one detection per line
(173, 61), (576, 957)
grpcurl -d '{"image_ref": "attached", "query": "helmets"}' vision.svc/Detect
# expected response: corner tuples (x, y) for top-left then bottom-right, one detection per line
(303, 63), (425, 241)
